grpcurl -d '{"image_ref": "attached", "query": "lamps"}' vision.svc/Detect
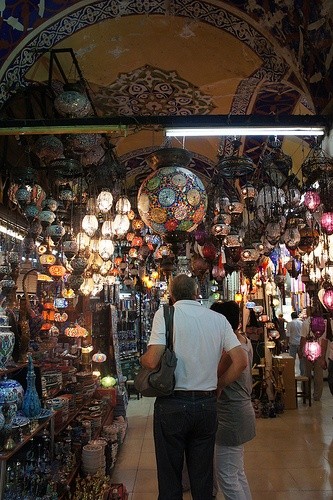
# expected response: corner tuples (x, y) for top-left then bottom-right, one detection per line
(0, 47), (333, 410)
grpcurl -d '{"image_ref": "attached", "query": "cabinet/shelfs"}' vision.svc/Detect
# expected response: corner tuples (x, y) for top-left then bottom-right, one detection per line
(0, 395), (116, 500)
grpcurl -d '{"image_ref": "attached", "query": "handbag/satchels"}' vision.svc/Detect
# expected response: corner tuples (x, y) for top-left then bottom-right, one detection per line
(133, 305), (178, 397)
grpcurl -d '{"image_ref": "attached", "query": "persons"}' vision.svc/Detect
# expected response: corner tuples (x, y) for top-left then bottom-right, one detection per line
(210, 300), (257, 500)
(278, 313), (288, 330)
(286, 312), (304, 376)
(140, 274), (247, 500)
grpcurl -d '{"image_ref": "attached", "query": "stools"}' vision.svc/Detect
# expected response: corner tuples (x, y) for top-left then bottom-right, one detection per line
(295, 374), (312, 408)
